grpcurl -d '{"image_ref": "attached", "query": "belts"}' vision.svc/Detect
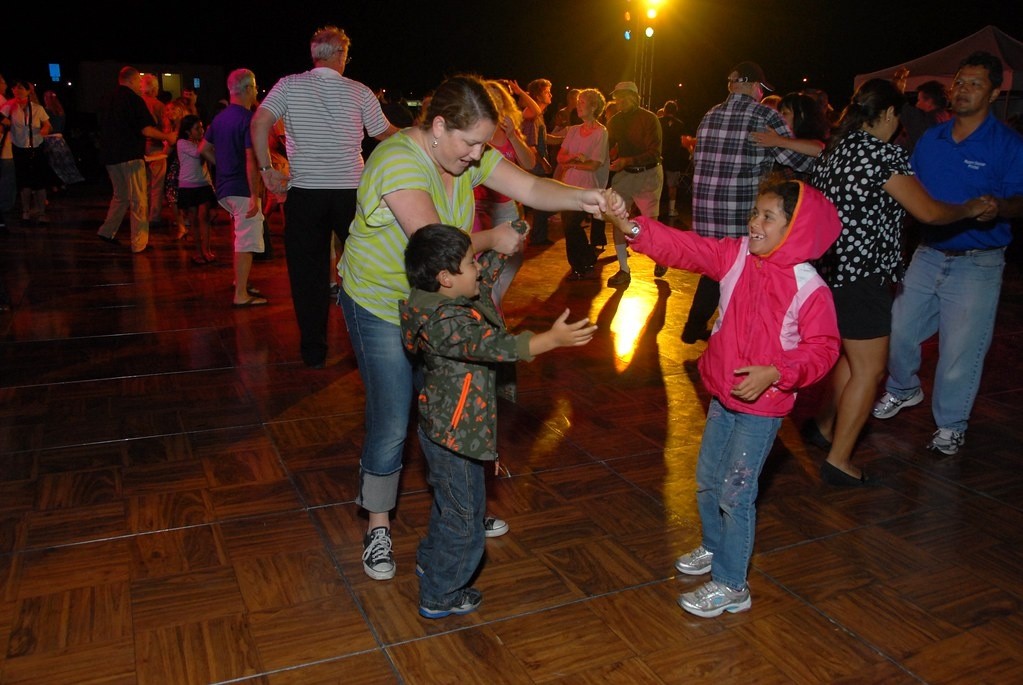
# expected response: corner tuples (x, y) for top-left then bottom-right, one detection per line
(936, 249), (983, 257)
(624, 161), (661, 174)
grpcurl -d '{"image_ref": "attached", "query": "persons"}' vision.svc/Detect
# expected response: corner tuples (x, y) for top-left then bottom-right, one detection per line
(681, 59), (1023, 345)
(95, 65), (414, 307)
(0, 74), (65, 230)
(398, 220), (598, 617)
(814, 77), (992, 487)
(601, 178), (842, 617)
(337, 76), (629, 579)
(412, 79), (685, 285)
(871, 51), (1023, 454)
(250, 27), (403, 369)
(556, 88), (609, 282)
(605, 82), (668, 285)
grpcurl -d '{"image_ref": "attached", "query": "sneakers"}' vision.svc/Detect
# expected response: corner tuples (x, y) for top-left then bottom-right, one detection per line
(677, 580), (752, 618)
(870, 387), (923, 419)
(419, 586), (484, 619)
(928, 427), (965, 456)
(483, 515), (510, 538)
(362, 527), (395, 579)
(674, 545), (715, 575)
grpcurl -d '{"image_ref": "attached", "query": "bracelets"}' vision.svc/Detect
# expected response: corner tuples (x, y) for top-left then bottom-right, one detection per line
(259, 164), (273, 171)
(625, 225), (639, 238)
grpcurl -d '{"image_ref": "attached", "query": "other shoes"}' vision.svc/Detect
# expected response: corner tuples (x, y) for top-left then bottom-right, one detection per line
(608, 270), (631, 285)
(566, 270), (586, 280)
(820, 460), (877, 490)
(682, 325), (711, 343)
(330, 282), (341, 297)
(584, 264), (596, 273)
(805, 417), (833, 453)
(0, 183), (283, 309)
(668, 210), (680, 216)
(528, 237), (556, 246)
(654, 263), (667, 277)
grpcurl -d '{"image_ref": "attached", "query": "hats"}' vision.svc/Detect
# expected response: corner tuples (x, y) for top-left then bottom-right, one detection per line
(727, 61), (775, 92)
(608, 82), (639, 97)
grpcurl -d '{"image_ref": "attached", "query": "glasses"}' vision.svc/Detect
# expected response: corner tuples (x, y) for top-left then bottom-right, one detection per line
(342, 54), (352, 64)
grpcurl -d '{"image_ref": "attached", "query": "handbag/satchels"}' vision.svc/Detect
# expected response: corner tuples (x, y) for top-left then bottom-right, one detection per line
(28, 148), (46, 177)
(165, 152), (182, 203)
